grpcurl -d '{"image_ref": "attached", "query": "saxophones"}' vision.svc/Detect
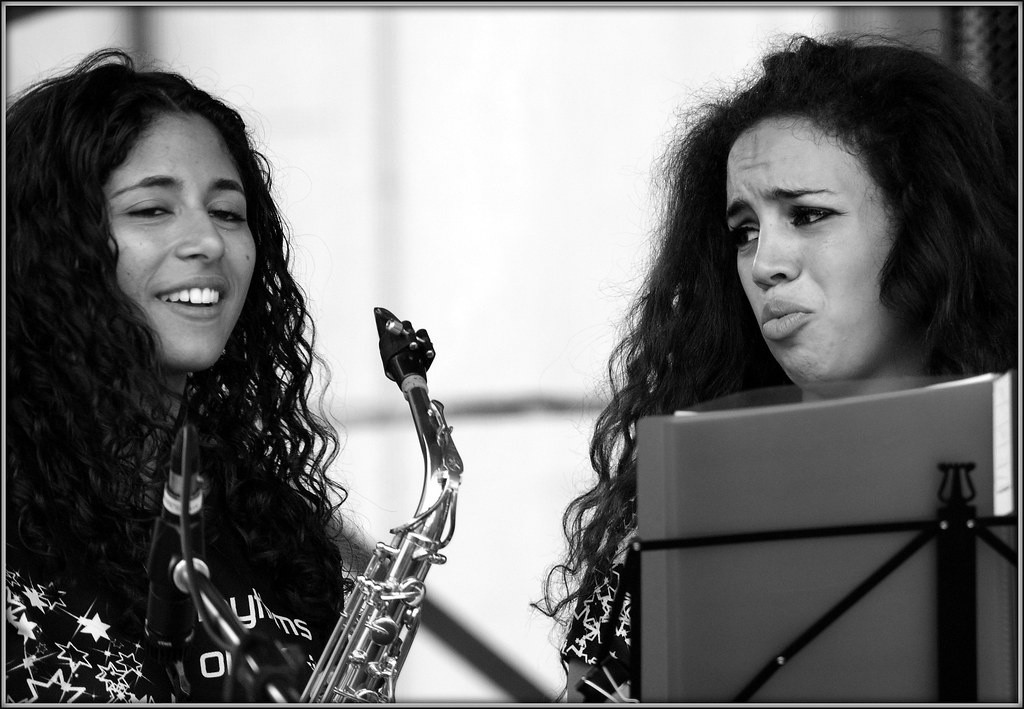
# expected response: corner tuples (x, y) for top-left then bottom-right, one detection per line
(298, 306), (466, 709)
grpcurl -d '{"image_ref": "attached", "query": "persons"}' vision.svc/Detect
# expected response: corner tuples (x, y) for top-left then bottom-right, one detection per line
(543, 33), (1017, 706)
(4, 48), (351, 708)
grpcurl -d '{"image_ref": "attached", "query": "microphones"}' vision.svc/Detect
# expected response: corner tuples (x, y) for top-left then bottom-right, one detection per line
(146, 425), (205, 645)
